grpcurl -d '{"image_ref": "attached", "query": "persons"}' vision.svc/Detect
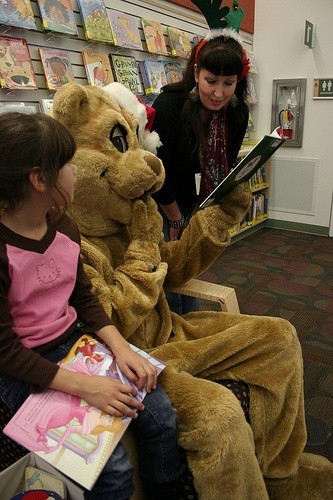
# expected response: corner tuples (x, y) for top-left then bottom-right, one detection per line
(150, 27), (250, 244)
(0, 112), (187, 500)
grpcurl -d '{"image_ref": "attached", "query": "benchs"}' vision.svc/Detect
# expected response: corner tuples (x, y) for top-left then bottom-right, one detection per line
(0, 279), (251, 500)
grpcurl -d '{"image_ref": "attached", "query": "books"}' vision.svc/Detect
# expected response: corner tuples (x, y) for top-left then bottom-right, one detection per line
(38, 0), (79, 37)
(139, 60), (183, 95)
(228, 162), (268, 235)
(82, 50), (114, 87)
(167, 29), (191, 58)
(142, 20), (167, 53)
(0, 0), (36, 30)
(4, 330), (169, 491)
(0, 36), (39, 90)
(197, 122), (285, 209)
(25, 465), (67, 500)
(40, 99), (54, 117)
(39, 48), (74, 92)
(112, 56), (144, 95)
(106, 9), (142, 51)
(80, 0), (113, 45)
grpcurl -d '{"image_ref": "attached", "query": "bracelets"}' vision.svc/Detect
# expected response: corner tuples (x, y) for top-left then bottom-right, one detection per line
(169, 216), (185, 229)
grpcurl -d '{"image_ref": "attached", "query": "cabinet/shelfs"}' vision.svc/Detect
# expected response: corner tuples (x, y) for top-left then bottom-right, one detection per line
(1, 0), (269, 246)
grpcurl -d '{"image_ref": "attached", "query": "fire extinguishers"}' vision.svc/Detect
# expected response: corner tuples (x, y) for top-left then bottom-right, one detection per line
(278, 104), (294, 139)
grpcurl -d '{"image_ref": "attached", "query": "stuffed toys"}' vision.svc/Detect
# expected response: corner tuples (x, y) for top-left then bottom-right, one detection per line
(53, 81), (333, 500)
